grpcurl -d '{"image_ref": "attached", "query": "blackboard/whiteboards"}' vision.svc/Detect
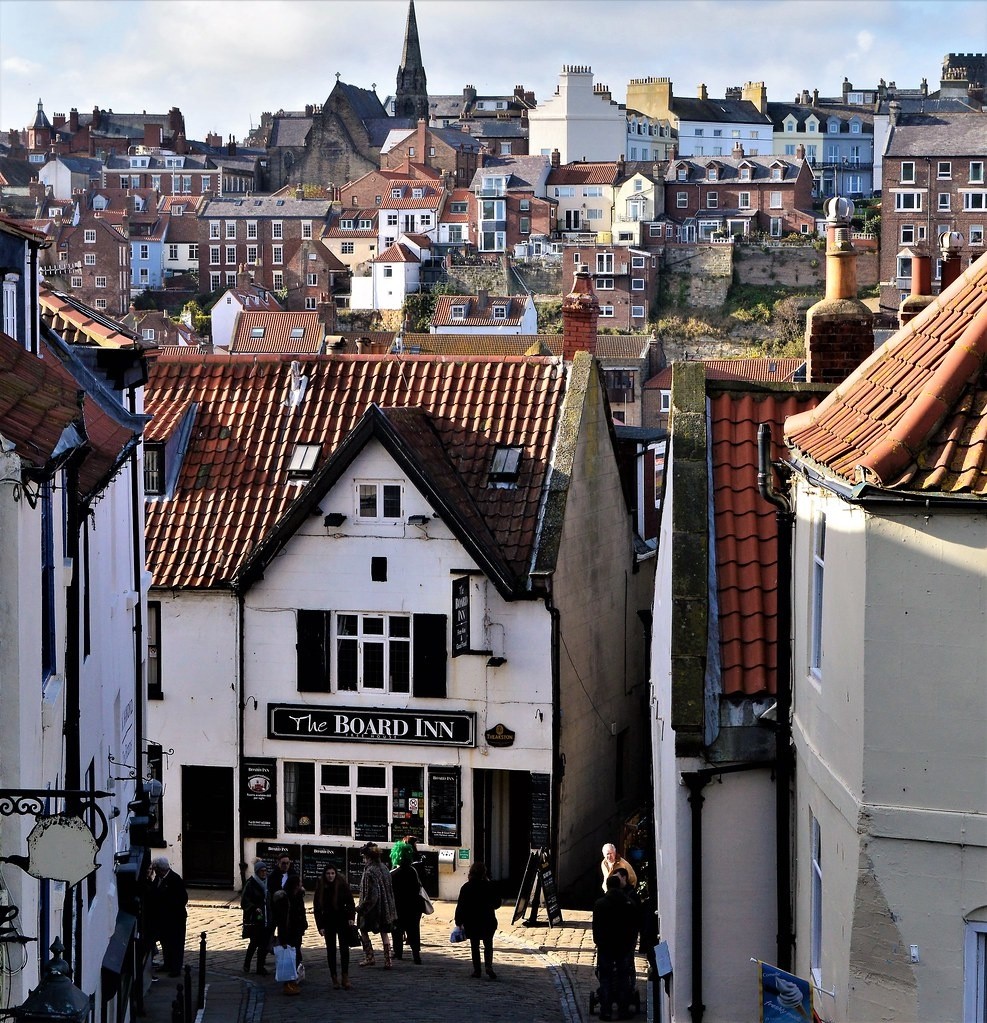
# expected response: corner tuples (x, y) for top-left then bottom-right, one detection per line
(539, 849), (561, 920)
(354, 821), (389, 842)
(391, 822), (424, 844)
(301, 844), (346, 892)
(255, 841), (301, 892)
(345, 846), (440, 898)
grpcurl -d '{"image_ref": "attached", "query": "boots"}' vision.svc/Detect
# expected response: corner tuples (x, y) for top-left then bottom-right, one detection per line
(358, 941), (375, 967)
(383, 943), (392, 969)
(341, 973), (352, 988)
(330, 973), (342, 989)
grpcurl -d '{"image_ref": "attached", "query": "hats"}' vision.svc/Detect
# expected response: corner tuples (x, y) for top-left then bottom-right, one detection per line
(254, 860), (267, 873)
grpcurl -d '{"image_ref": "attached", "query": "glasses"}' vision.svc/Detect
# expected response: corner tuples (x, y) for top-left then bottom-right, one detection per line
(278, 861), (290, 866)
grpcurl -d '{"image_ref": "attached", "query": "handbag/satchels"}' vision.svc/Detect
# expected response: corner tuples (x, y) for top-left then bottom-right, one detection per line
(348, 925), (363, 947)
(273, 944), (298, 981)
(450, 925), (467, 943)
(411, 866), (434, 915)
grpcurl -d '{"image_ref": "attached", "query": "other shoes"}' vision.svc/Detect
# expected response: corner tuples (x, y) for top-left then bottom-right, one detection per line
(151, 975), (159, 981)
(152, 963), (159, 967)
(286, 983), (301, 992)
(393, 952), (402, 960)
(485, 971), (497, 980)
(413, 959), (422, 965)
(168, 970), (181, 978)
(471, 971), (482, 978)
(257, 969), (271, 975)
(158, 965), (171, 972)
(243, 966), (250, 973)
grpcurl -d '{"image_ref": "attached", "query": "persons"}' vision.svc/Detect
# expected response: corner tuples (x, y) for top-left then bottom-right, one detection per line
(355, 842), (398, 968)
(313, 864), (356, 988)
(145, 859), (188, 977)
(455, 862), (502, 979)
(593, 876), (638, 1021)
(601, 843), (641, 991)
(241, 853), (308, 993)
(387, 835), (428, 964)
